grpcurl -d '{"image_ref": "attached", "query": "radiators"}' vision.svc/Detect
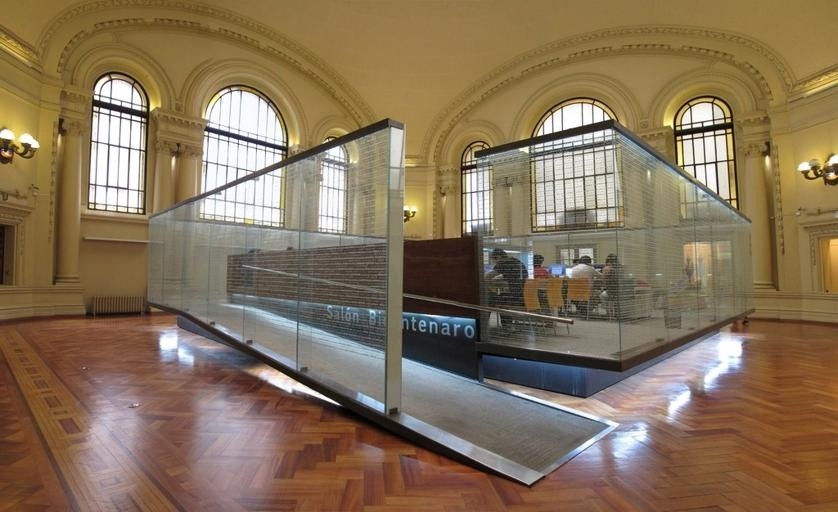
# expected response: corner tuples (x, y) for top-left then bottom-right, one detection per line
(91, 295), (145, 317)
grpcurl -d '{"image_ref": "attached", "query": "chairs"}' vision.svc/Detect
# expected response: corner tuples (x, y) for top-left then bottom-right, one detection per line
(496, 277), (635, 337)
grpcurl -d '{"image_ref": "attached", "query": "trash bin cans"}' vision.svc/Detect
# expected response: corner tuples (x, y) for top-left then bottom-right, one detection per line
(663, 296), (682, 328)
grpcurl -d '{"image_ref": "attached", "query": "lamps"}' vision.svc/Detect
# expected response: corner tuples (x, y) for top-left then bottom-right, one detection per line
(0, 128), (40, 164)
(798, 153), (838, 187)
(404, 206), (417, 223)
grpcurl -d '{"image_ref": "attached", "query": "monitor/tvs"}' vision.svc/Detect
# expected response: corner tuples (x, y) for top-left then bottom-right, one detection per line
(478, 250), (605, 283)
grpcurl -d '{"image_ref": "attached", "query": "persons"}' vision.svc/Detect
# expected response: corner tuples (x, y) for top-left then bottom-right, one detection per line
(572, 258), (580, 268)
(599, 252), (623, 321)
(532, 253), (551, 303)
(570, 255), (602, 316)
(484, 246), (528, 335)
